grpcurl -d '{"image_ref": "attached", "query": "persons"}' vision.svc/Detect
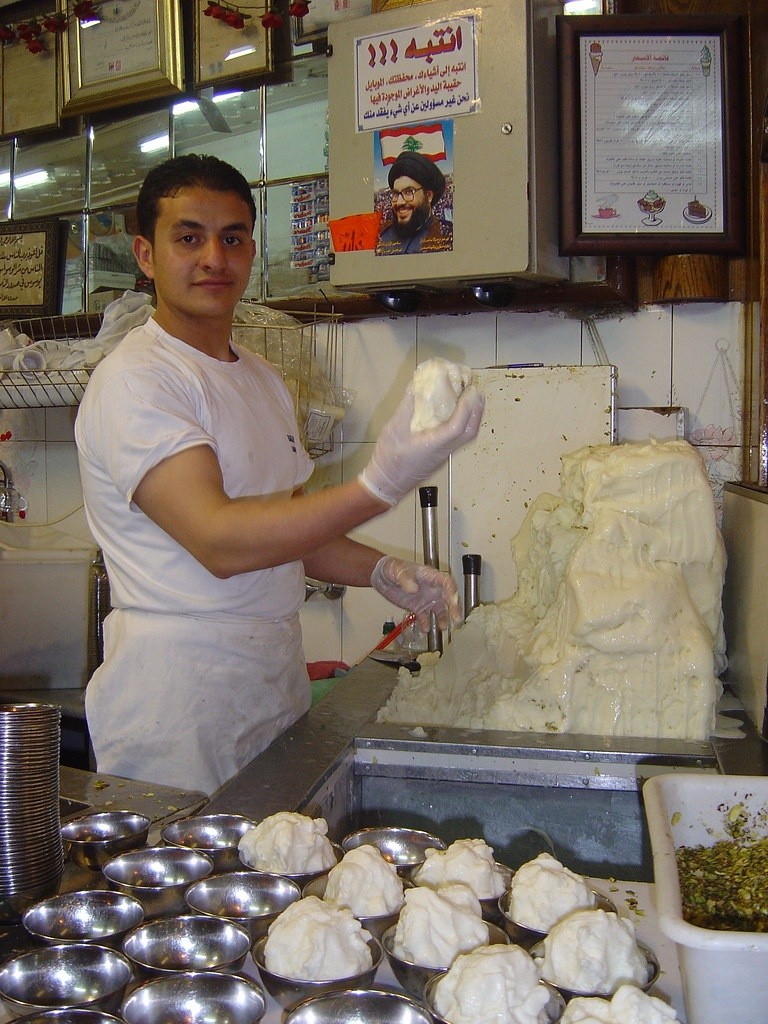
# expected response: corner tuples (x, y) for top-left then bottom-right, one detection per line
(75, 152), (486, 797)
(375, 151), (453, 256)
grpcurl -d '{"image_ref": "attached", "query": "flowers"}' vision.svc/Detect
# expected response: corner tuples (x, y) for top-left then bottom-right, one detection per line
(202, 0), (312, 30)
(0, 0), (99, 54)
(204, 0), (313, 31)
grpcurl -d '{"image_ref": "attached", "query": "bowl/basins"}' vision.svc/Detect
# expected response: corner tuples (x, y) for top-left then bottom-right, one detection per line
(529, 938), (660, 1004)
(122, 915), (251, 983)
(239, 836), (345, 889)
(62, 810), (150, 871)
(121, 969), (268, 1024)
(305, 871), (416, 938)
(101, 846), (214, 921)
(0, 702), (63, 921)
(185, 872), (302, 949)
(251, 929), (385, 1011)
(410, 857), (517, 924)
(0, 944), (126, 1024)
(284, 988), (434, 1024)
(381, 918), (567, 1024)
(343, 827), (448, 881)
(499, 886), (618, 953)
(161, 814), (257, 872)
(23, 889), (144, 950)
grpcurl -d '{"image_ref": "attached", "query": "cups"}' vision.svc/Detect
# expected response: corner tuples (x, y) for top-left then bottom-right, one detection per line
(0, 328), (18, 369)
(14, 350), (48, 379)
(85, 351), (106, 371)
(16, 333), (34, 346)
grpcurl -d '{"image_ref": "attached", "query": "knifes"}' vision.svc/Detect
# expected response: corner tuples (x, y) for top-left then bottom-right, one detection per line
(369, 649), (422, 670)
(486, 362), (544, 368)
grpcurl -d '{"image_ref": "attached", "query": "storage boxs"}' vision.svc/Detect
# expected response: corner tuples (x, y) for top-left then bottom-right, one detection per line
(642, 773), (768, 1024)
(89, 285), (143, 314)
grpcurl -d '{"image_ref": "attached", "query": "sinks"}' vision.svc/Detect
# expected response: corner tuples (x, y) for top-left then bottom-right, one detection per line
(290, 733), (730, 885)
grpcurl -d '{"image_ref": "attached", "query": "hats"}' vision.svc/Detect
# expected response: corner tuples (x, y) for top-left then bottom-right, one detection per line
(388, 151), (445, 207)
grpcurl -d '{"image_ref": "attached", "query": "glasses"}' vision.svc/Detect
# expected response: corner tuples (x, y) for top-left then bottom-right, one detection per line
(390, 187), (423, 202)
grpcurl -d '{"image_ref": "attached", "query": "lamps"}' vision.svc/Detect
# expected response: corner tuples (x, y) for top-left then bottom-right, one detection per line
(14, 166), (54, 190)
(137, 129), (169, 153)
